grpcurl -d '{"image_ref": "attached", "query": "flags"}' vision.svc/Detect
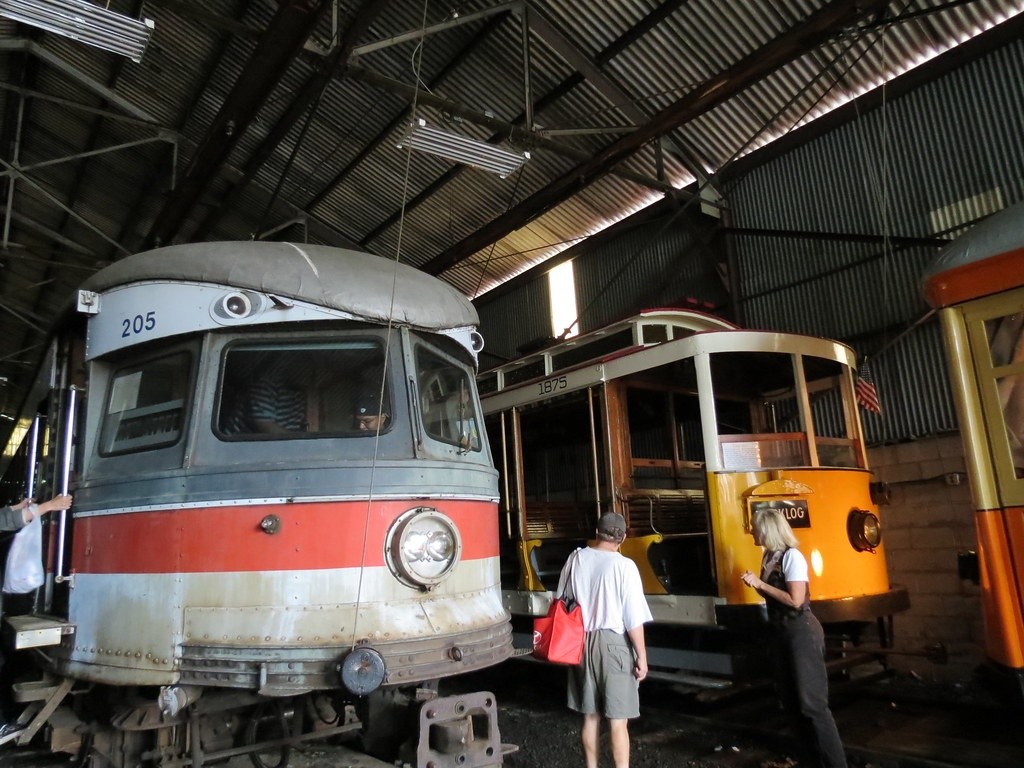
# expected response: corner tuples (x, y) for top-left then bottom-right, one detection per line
(856, 362), (882, 415)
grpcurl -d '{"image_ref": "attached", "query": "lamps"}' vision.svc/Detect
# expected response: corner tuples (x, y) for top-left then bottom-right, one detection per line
(0, 0), (155, 64)
(396, 98), (531, 180)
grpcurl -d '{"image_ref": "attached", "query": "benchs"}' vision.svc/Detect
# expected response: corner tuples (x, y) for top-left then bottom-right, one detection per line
(619, 489), (709, 595)
(522, 501), (599, 592)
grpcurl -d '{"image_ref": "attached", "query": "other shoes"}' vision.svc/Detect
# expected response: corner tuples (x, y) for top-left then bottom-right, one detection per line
(0, 723), (27, 745)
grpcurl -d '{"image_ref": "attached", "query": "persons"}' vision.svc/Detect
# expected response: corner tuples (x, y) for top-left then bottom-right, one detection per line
(555, 511), (655, 768)
(0, 493), (73, 746)
(740, 508), (849, 767)
(355, 395), (387, 432)
(221, 351), (323, 438)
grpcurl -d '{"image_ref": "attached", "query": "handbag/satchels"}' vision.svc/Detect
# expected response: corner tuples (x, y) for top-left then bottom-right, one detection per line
(530, 549), (584, 664)
(2, 504), (44, 594)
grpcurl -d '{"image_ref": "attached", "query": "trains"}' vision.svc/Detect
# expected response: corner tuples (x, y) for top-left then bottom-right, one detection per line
(425, 306), (914, 715)
(0, 238), (531, 768)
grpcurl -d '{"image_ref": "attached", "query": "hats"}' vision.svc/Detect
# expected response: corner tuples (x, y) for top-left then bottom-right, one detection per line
(354, 398), (383, 416)
(598, 513), (626, 538)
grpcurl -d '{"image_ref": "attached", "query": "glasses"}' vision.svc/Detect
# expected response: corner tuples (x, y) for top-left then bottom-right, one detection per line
(356, 417), (377, 425)
